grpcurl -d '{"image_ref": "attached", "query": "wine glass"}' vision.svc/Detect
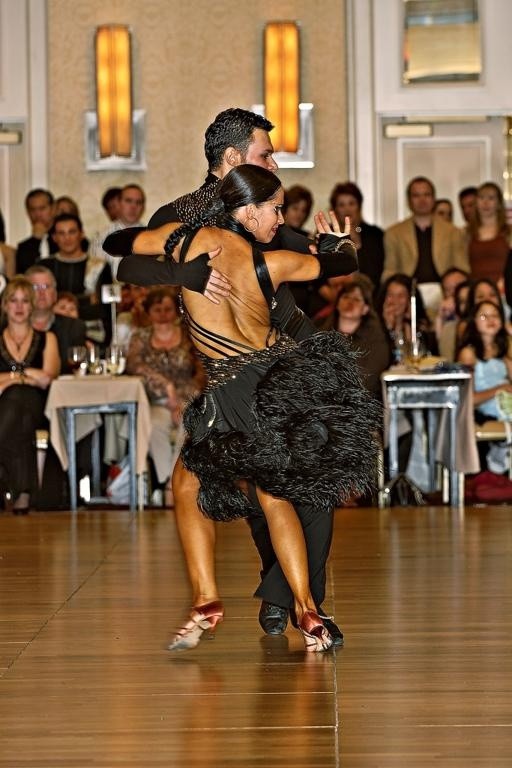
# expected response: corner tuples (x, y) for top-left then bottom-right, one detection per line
(106, 345), (123, 378)
(392, 335), (408, 369)
(86, 346), (101, 376)
(68, 346), (87, 378)
(405, 342), (426, 375)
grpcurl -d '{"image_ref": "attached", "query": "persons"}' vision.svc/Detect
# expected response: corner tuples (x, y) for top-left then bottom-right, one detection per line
(102, 164), (358, 656)
(0, 182), (208, 516)
(277, 178), (512, 505)
(114, 106), (332, 653)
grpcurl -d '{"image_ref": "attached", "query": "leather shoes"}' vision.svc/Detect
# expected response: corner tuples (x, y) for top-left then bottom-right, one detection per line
(259, 600), (289, 638)
(316, 608), (345, 647)
(12, 505), (30, 516)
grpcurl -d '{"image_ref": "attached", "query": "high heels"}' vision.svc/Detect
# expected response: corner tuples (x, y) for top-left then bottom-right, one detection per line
(299, 612), (334, 653)
(167, 600), (226, 653)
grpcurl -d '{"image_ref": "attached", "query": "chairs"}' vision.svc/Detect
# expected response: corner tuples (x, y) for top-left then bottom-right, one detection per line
(38, 430), (67, 510)
(377, 442), (385, 510)
(460, 433), (510, 511)
(137, 428), (189, 512)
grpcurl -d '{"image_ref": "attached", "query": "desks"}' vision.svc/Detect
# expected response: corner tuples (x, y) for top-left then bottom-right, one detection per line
(45, 369), (152, 513)
(381, 362), (480, 510)
